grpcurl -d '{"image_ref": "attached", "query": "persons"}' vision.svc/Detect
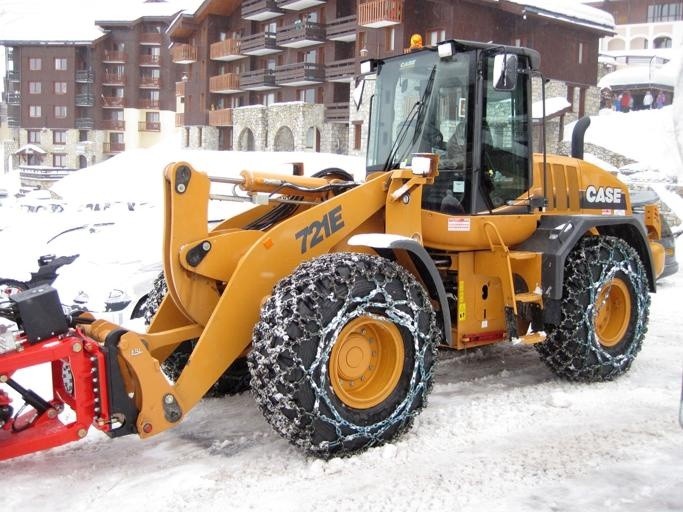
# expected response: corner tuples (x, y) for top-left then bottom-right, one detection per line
(611, 90), (666, 112)
(447, 86), (493, 166)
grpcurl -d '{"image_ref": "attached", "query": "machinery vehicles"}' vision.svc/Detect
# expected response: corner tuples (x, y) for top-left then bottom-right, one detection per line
(61, 31), (681, 464)
